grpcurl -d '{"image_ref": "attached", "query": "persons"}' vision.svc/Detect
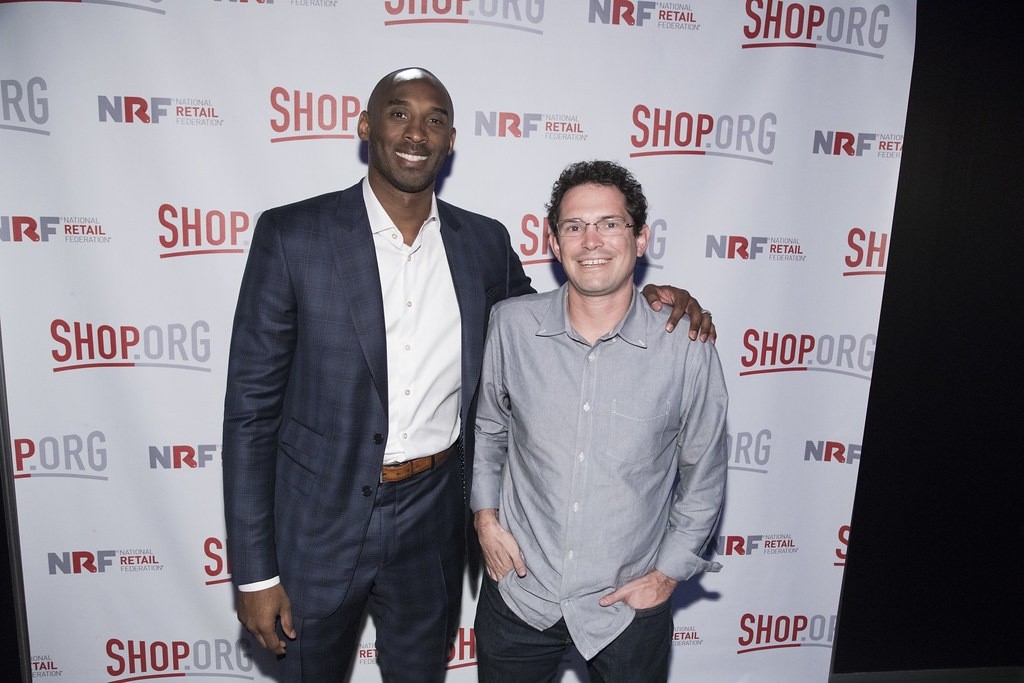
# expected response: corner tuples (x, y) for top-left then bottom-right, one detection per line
(220, 65), (719, 683)
(468, 157), (731, 683)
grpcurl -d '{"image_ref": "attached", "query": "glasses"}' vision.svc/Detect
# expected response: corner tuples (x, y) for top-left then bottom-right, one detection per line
(554, 217), (636, 237)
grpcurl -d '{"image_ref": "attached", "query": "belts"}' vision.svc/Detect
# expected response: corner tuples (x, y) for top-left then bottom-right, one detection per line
(377, 446), (448, 484)
